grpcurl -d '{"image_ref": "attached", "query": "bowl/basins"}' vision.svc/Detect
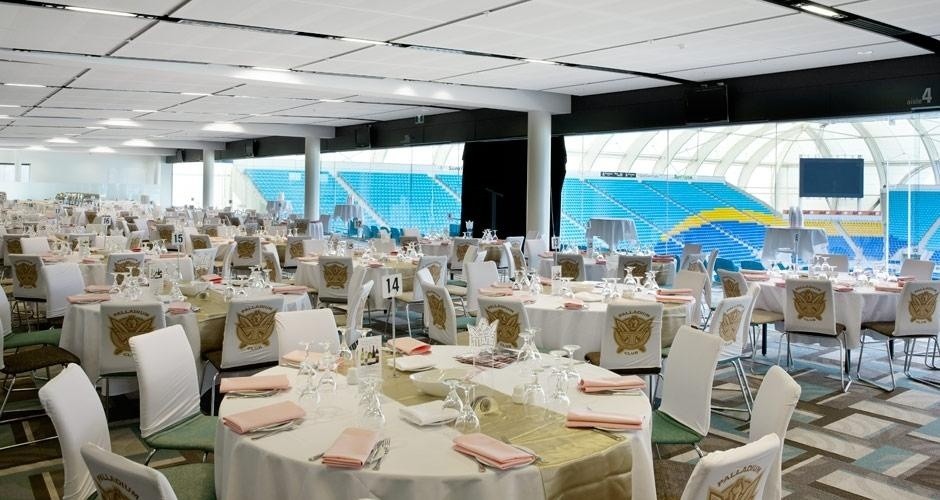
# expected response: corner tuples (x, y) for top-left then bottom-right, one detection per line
(411, 369), (472, 398)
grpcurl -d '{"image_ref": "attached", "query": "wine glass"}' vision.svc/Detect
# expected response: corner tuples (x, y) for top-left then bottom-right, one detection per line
(442, 326), (583, 435)
(47, 238), (171, 258)
(112, 264), (274, 302)
(298, 324), (386, 431)
(323, 238), (422, 268)
(430, 227), (501, 243)
(2, 193), (223, 236)
(222, 224), (299, 242)
(763, 255), (896, 287)
(495, 265), (660, 311)
(558, 236), (650, 261)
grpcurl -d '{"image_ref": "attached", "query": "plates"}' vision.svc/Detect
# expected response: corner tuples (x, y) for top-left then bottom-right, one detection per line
(229, 388), (276, 399)
(429, 418), (454, 428)
(251, 420), (294, 434)
(474, 444), (536, 470)
(606, 388), (640, 398)
(593, 425), (632, 433)
(388, 354), (436, 373)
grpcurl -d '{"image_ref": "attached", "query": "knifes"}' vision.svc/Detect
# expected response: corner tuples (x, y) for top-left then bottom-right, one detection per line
(366, 440), (383, 464)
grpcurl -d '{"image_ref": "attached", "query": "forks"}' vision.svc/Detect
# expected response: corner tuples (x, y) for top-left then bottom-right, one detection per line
(374, 438), (393, 471)
(500, 435), (549, 466)
(252, 421), (306, 439)
